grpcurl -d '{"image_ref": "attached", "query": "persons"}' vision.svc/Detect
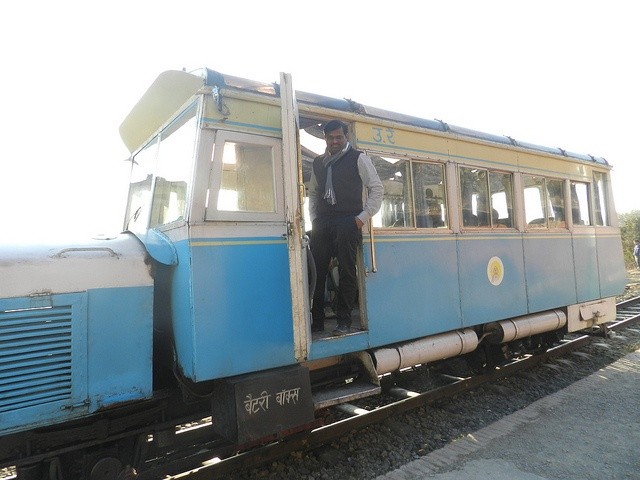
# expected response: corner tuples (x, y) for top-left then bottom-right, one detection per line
(424, 189), (442, 228)
(308, 119), (384, 339)
(634, 240), (640, 269)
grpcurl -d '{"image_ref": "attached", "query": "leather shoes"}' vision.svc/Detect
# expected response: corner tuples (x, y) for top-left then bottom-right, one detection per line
(311, 324), (324, 332)
(336, 320), (350, 333)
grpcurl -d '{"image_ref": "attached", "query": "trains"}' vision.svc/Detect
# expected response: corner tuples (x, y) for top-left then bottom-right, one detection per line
(1, 67), (626, 480)
